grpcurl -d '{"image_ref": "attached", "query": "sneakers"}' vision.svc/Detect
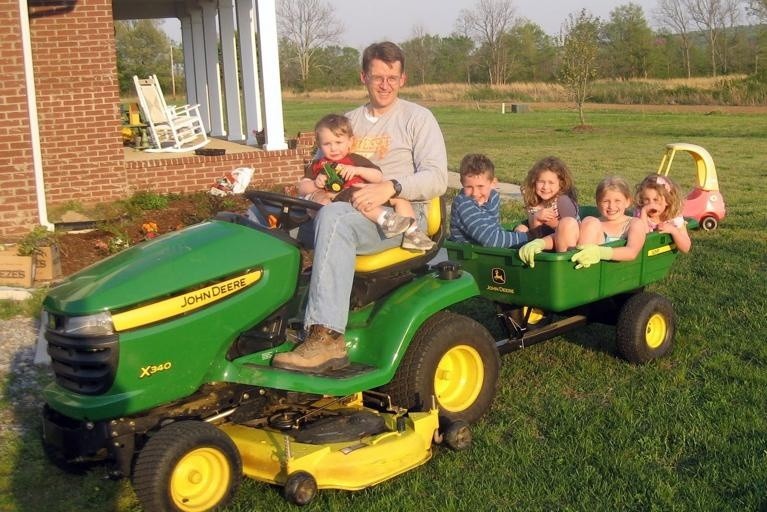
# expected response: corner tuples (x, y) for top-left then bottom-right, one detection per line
(379, 210), (413, 239)
(402, 228), (438, 251)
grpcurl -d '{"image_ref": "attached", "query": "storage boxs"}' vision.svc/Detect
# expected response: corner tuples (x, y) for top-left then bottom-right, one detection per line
(5, 244), (61, 280)
(0, 251), (35, 289)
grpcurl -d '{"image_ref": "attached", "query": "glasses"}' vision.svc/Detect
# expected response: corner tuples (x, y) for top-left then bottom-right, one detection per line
(370, 75), (400, 85)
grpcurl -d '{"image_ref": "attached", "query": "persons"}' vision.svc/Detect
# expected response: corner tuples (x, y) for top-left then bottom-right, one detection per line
(632, 172), (691, 255)
(520, 178), (646, 271)
(272, 42), (447, 373)
(514, 155), (581, 233)
(295, 113), (439, 250)
(449, 154), (559, 249)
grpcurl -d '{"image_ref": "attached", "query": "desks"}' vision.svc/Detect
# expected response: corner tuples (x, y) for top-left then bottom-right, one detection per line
(127, 123), (158, 149)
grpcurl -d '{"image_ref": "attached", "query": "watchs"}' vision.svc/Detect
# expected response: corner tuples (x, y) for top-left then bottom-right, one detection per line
(389, 178), (402, 197)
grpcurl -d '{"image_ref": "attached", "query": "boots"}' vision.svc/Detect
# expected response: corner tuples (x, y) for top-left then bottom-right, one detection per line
(272, 325), (350, 373)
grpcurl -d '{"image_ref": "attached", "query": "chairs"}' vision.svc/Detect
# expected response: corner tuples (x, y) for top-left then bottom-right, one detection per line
(133, 74), (211, 153)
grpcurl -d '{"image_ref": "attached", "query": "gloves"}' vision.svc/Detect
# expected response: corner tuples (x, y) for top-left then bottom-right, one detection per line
(519, 238), (545, 268)
(571, 244), (613, 270)
